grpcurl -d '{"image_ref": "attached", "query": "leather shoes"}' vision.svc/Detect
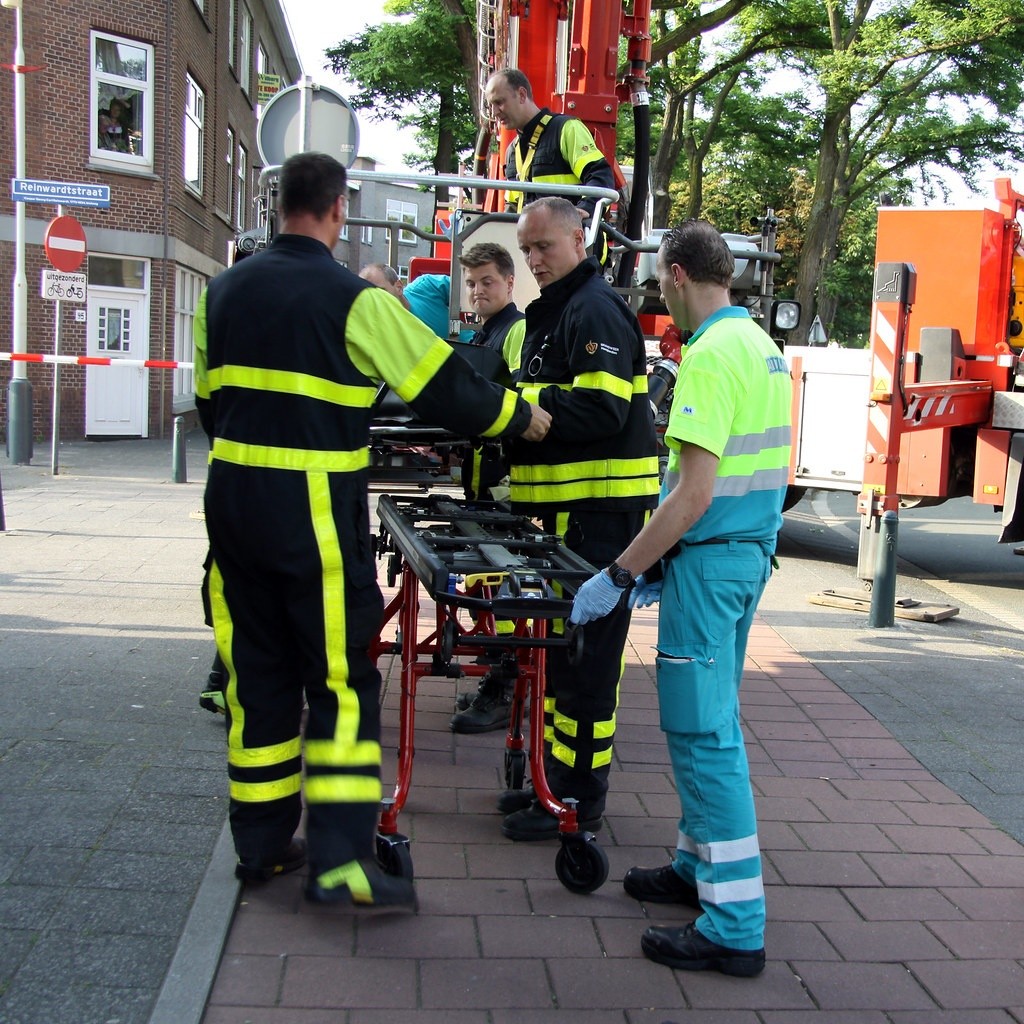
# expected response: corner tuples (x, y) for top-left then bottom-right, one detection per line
(642, 922), (765, 978)
(624, 863), (701, 909)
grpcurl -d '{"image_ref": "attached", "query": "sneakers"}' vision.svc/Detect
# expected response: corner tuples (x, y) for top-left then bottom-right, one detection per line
(305, 860), (415, 916)
(234, 838), (306, 879)
(497, 779), (537, 811)
(456, 691), (530, 719)
(450, 680), (514, 735)
(199, 680), (225, 715)
(501, 799), (603, 841)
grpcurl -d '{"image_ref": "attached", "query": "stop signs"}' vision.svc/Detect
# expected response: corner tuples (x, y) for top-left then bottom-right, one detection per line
(45, 215), (87, 273)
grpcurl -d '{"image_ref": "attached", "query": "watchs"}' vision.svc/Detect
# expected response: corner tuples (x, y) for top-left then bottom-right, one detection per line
(605, 560), (639, 590)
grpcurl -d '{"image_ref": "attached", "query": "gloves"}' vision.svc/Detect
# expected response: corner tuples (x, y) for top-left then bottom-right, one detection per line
(570, 569), (625, 625)
(628, 559), (668, 609)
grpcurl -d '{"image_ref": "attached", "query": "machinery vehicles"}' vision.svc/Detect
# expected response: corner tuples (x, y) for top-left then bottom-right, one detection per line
(406, 0), (1024, 632)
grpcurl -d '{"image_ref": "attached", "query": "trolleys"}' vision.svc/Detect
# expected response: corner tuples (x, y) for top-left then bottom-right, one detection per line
(371, 489), (612, 894)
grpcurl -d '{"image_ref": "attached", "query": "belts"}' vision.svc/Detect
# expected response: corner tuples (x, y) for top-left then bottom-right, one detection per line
(684, 538), (761, 548)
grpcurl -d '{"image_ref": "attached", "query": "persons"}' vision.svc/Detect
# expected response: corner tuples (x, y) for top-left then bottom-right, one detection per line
(356, 261), (459, 346)
(569, 220), (794, 975)
(487, 196), (662, 847)
(481, 69), (623, 272)
(452, 245), (528, 734)
(98, 99), (133, 154)
(190, 150), (553, 914)
